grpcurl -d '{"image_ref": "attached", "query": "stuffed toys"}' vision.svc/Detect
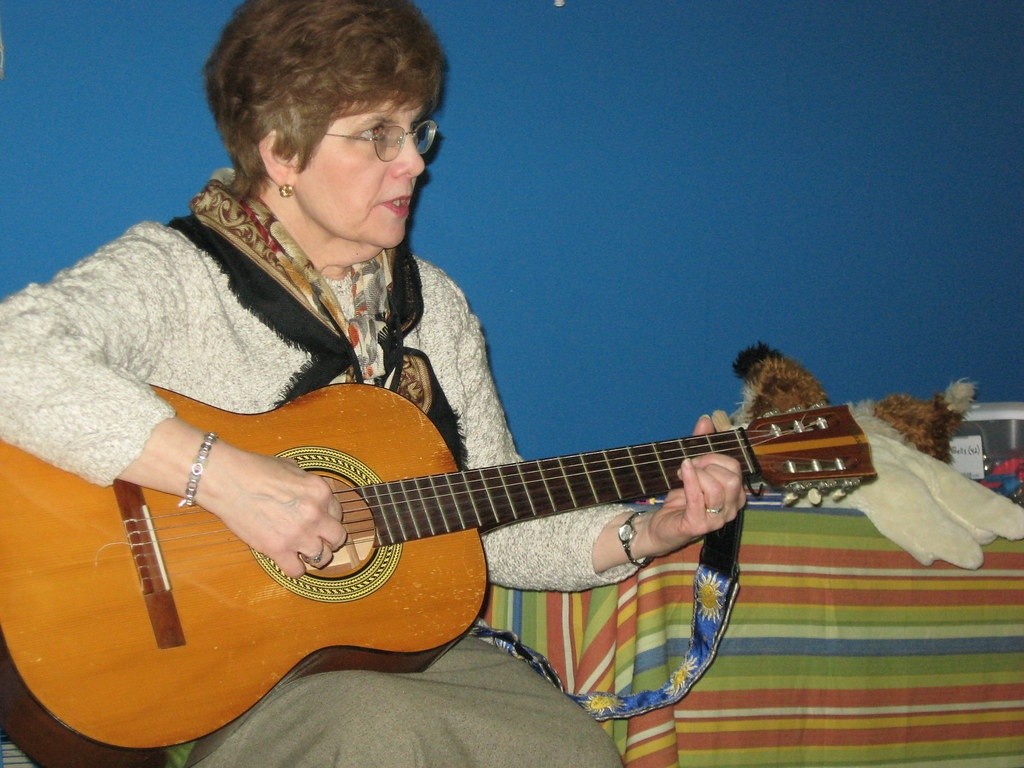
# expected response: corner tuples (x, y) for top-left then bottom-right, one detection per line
(730, 340), (977, 470)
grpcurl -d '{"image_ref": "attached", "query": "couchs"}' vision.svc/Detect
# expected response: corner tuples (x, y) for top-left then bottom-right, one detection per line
(0, 451), (1024, 768)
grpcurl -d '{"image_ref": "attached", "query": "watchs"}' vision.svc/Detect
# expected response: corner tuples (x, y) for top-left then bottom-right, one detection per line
(618, 511), (655, 567)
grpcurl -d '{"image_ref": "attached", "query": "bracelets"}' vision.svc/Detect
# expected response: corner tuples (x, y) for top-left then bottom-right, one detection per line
(185, 432), (218, 507)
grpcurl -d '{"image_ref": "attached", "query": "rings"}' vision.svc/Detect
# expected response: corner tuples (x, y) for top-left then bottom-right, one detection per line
(302, 541), (325, 563)
(705, 501), (725, 516)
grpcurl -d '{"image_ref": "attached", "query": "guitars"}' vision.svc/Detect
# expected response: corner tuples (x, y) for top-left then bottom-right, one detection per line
(0, 382), (880, 768)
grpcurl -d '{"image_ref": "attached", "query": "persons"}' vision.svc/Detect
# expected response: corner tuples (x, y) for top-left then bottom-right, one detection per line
(1, 1), (748, 768)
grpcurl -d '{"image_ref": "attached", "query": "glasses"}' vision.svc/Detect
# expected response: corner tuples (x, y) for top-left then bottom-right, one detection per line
(325, 120), (439, 162)
